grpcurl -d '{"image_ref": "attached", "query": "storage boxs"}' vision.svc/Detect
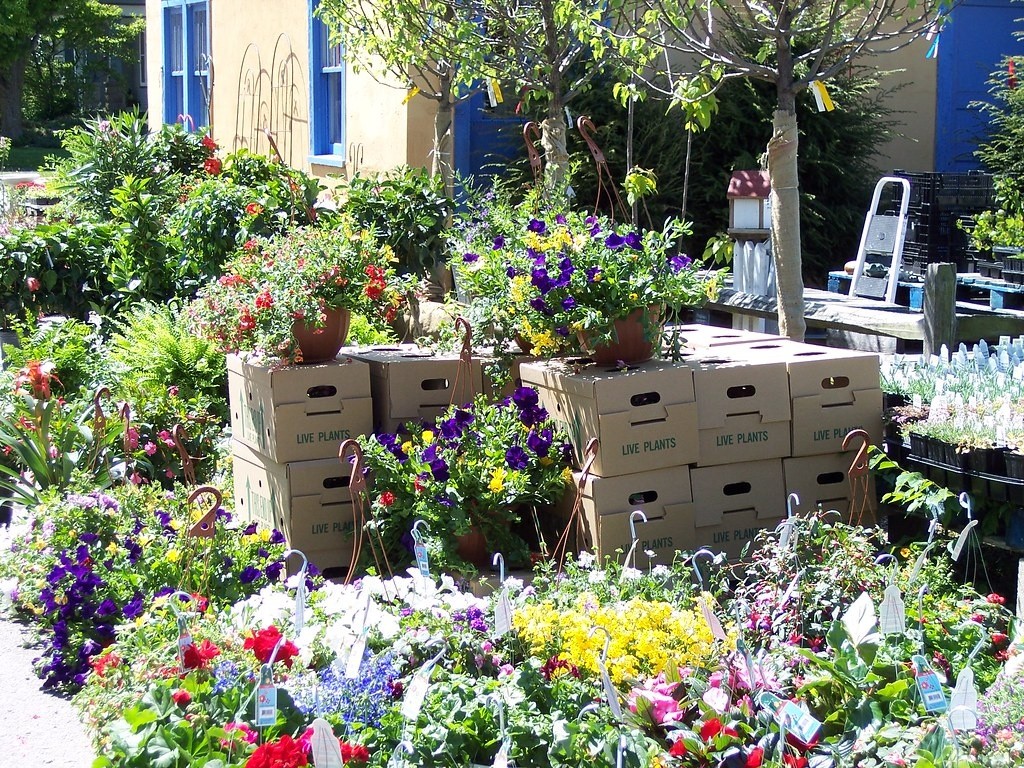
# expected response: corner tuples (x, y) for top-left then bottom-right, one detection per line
(222, 324), (882, 580)
(892, 167), (1020, 285)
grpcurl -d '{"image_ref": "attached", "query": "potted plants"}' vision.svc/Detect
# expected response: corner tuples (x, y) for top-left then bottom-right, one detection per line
(952, 16), (1024, 286)
(876, 334), (1024, 504)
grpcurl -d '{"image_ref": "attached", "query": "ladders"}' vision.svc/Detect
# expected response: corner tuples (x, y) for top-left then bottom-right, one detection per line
(847, 176), (911, 304)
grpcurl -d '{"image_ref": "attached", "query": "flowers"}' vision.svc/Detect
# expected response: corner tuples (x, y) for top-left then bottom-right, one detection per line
(438, 162), (735, 368)
(173, 215), (434, 373)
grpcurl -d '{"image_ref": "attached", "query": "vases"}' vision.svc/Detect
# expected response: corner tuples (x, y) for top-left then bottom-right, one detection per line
(577, 302), (663, 367)
(284, 306), (353, 364)
(512, 328), (537, 354)
